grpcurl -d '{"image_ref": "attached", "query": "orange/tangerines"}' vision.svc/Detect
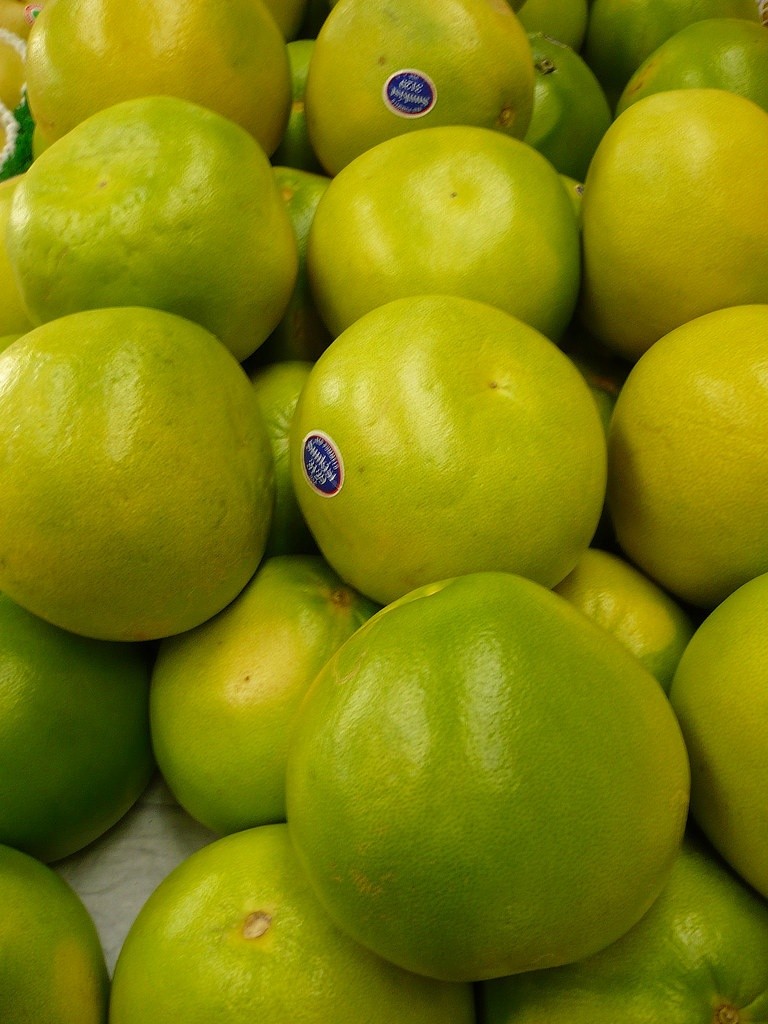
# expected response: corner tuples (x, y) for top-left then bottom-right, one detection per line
(0, 0), (767, 1020)
